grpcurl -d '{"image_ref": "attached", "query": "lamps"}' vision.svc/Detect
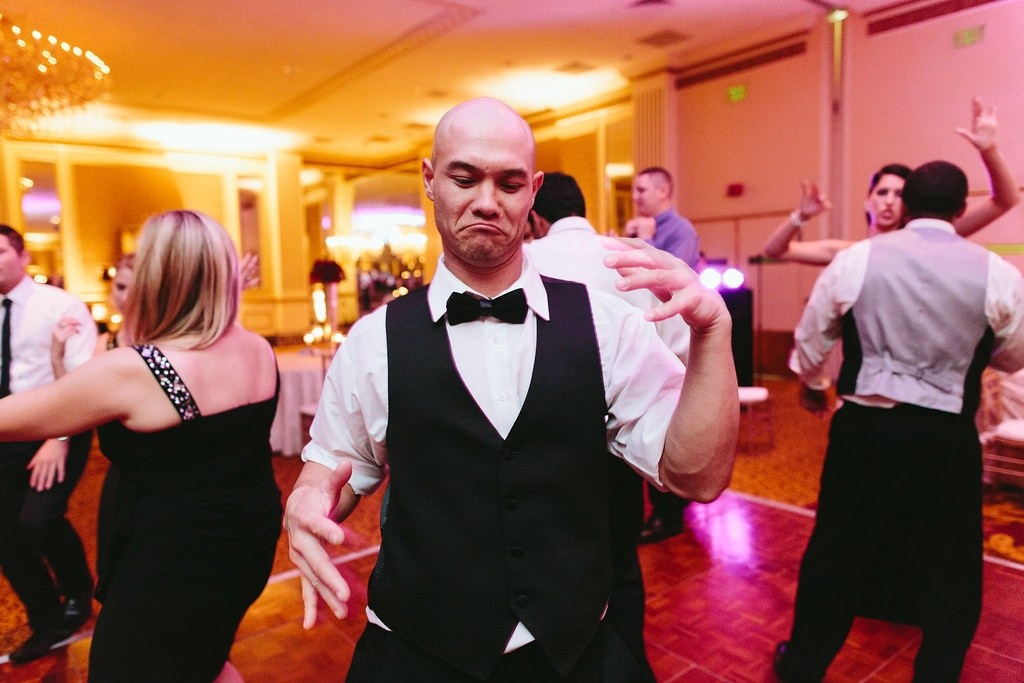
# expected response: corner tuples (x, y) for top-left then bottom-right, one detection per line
(0, 14), (114, 139)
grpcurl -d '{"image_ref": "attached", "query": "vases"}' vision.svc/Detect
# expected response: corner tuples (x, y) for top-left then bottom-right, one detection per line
(324, 284), (337, 329)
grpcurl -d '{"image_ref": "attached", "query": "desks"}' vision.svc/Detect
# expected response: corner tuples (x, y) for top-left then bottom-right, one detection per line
(270, 346), (334, 456)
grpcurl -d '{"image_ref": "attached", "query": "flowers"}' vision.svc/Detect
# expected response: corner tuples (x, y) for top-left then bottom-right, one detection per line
(310, 257), (345, 285)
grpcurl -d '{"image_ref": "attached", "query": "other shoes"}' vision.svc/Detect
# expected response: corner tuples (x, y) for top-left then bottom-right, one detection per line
(42, 610), (94, 651)
(637, 505), (684, 543)
(775, 643), (788, 672)
(8, 627), (57, 665)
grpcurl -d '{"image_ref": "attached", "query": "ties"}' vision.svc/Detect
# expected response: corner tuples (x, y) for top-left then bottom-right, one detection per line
(0, 299), (12, 399)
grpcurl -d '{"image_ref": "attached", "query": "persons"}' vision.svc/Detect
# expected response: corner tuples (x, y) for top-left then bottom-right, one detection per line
(283, 97), (738, 682)
(626, 167), (698, 543)
(0, 209), (283, 683)
(758, 97), (1023, 683)
(521, 172), (690, 369)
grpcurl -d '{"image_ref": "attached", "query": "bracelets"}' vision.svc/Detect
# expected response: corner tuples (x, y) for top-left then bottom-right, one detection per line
(58, 437), (70, 440)
(791, 214), (803, 227)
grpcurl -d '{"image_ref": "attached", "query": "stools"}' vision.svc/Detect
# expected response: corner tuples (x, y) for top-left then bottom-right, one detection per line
(739, 387), (775, 455)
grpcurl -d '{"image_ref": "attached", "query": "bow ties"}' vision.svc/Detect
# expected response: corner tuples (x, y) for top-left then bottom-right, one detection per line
(446, 288), (529, 326)
